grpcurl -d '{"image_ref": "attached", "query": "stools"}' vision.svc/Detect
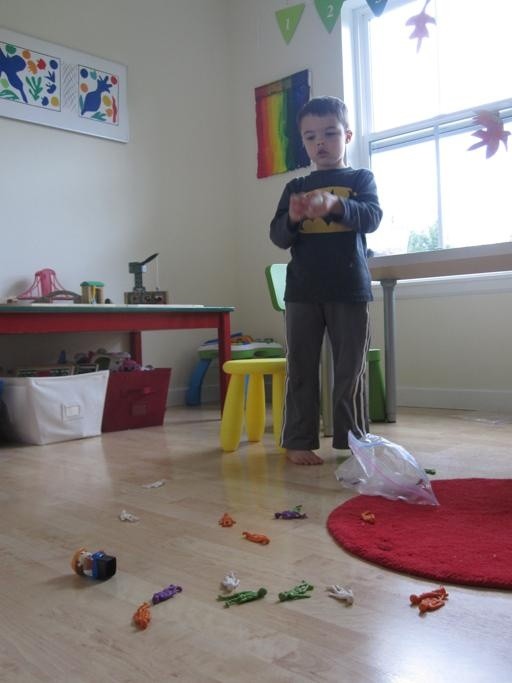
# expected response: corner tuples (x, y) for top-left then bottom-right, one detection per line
(187, 341), (285, 407)
(219, 358), (291, 455)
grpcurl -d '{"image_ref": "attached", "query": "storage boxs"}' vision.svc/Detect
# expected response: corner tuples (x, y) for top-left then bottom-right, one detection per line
(102, 367), (172, 433)
(0, 369), (110, 445)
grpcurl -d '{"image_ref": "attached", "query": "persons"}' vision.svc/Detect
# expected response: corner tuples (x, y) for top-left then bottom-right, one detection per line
(410, 585), (449, 614)
(133, 584), (182, 628)
(217, 570), (354, 607)
(119, 479), (166, 522)
(361, 511), (375, 522)
(267, 94), (384, 467)
(71, 547), (104, 578)
(218, 504), (307, 545)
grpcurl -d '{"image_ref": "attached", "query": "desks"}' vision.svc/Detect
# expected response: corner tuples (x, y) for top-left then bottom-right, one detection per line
(364, 241), (512, 422)
(0, 301), (235, 420)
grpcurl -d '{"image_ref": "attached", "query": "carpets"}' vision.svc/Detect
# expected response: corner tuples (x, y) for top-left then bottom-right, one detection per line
(327, 478), (512, 589)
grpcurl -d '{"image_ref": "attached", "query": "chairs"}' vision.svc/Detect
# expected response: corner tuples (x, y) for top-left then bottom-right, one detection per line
(265, 263), (387, 423)
